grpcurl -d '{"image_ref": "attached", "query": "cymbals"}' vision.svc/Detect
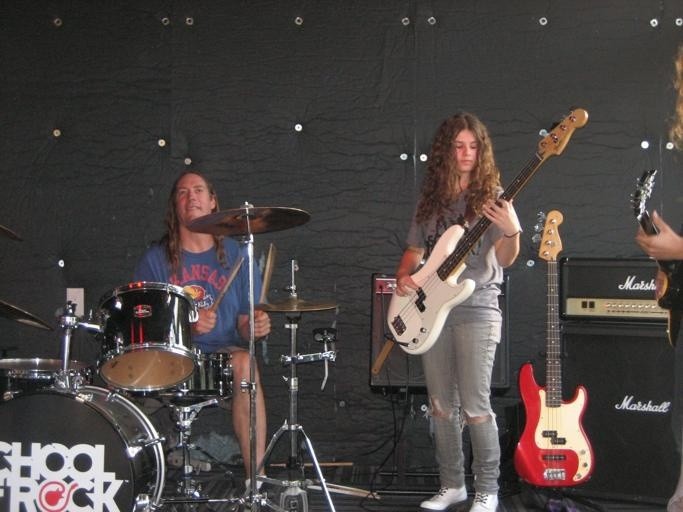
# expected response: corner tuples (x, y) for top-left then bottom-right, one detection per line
(0, 300), (53, 331)
(0, 224), (22, 241)
(254, 299), (340, 312)
(185, 206), (311, 237)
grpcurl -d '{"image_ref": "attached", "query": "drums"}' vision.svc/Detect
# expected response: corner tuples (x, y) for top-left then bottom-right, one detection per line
(0, 385), (165, 512)
(152, 352), (232, 402)
(0, 358), (89, 398)
(96, 281), (199, 393)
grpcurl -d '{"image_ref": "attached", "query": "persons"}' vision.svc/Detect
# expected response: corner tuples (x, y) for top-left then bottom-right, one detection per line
(396, 111), (523, 512)
(635, 45), (683, 512)
(133, 170), (271, 510)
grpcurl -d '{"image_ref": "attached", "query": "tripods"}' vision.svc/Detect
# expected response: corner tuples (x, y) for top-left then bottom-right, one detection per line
(218, 233), (286, 511)
(156, 397), (226, 506)
(233, 314), (334, 512)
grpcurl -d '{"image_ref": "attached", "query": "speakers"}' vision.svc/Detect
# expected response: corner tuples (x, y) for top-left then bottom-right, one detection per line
(557, 256), (681, 321)
(372, 273), (508, 393)
(560, 324), (683, 508)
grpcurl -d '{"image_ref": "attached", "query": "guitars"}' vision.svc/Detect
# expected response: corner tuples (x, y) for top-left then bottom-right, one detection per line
(514, 211), (595, 488)
(387, 107), (588, 354)
(630, 168), (683, 348)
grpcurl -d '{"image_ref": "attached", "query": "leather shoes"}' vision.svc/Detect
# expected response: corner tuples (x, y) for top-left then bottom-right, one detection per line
(421, 483), (468, 511)
(468, 493), (499, 512)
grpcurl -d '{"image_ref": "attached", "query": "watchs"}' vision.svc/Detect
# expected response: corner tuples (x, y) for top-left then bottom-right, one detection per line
(504, 228), (523, 238)
